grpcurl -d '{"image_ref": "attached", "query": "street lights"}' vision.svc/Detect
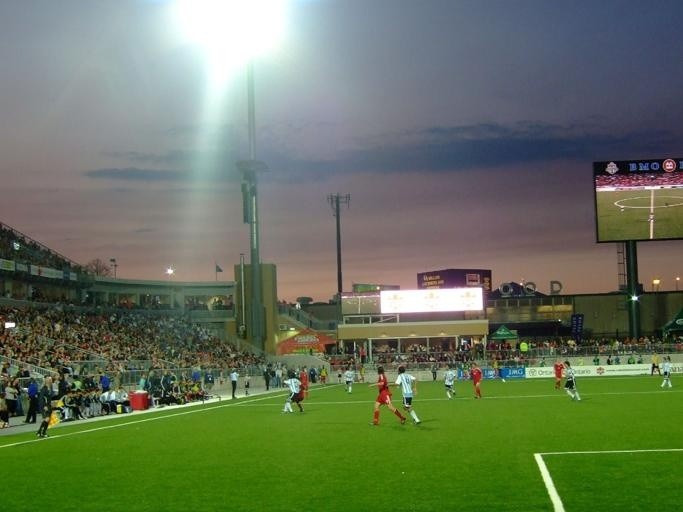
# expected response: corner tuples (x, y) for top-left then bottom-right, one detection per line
(241, 1), (267, 356)
(676, 276), (679, 291)
(327, 193), (352, 293)
(109, 258), (117, 280)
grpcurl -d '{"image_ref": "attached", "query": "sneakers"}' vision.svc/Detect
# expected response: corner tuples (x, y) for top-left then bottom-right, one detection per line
(570, 395), (581, 401)
(400, 416), (407, 425)
(344, 389), (353, 394)
(35, 431), (50, 438)
(281, 409), (305, 414)
(368, 420), (379, 426)
(413, 418), (421, 425)
(474, 393), (481, 399)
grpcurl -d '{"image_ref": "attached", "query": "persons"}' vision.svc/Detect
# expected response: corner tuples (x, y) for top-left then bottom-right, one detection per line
(620, 203), (625, 213)
(1, 214), (326, 439)
(596, 172), (683, 186)
(659, 356), (673, 387)
(552, 360), (564, 389)
(370, 367), (405, 426)
(616, 190), (619, 198)
(330, 334), (683, 383)
(343, 368), (354, 393)
(648, 212), (656, 222)
(469, 363), (482, 399)
(393, 368), (421, 424)
(563, 361), (580, 401)
(664, 201), (669, 208)
(443, 364), (455, 399)
